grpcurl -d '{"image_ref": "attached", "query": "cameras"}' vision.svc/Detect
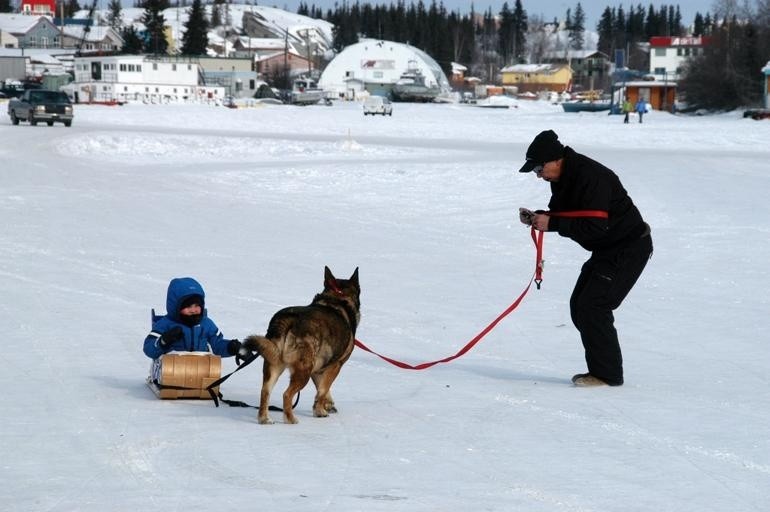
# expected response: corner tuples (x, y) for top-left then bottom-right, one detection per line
(522, 210), (534, 218)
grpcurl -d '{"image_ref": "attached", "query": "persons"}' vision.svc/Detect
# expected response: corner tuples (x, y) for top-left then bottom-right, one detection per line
(140, 276), (240, 357)
(634, 96), (645, 123)
(519, 131), (653, 387)
(622, 97), (634, 124)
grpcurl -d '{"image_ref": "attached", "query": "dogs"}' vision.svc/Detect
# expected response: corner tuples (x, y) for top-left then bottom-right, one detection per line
(242, 265), (361, 425)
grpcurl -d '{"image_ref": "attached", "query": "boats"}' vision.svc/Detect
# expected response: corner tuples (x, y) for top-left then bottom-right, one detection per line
(561, 97), (609, 114)
(390, 69), (442, 103)
(280, 73), (327, 106)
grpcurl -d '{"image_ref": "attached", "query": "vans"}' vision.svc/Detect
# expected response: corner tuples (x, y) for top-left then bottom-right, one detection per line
(362, 96), (393, 117)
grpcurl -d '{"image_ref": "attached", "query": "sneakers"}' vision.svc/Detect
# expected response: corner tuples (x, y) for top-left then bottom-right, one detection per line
(571, 371), (608, 387)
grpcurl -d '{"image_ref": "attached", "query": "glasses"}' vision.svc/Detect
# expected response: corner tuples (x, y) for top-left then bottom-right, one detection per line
(530, 161), (550, 176)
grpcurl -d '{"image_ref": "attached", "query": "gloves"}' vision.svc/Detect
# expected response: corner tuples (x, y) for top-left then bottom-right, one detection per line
(163, 326), (184, 345)
(227, 338), (241, 356)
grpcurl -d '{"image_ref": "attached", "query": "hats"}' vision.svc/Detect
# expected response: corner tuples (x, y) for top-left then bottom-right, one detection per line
(179, 294), (205, 327)
(517, 130), (567, 173)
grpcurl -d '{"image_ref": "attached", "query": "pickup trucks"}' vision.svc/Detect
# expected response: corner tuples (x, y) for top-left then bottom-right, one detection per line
(7, 89), (74, 129)
